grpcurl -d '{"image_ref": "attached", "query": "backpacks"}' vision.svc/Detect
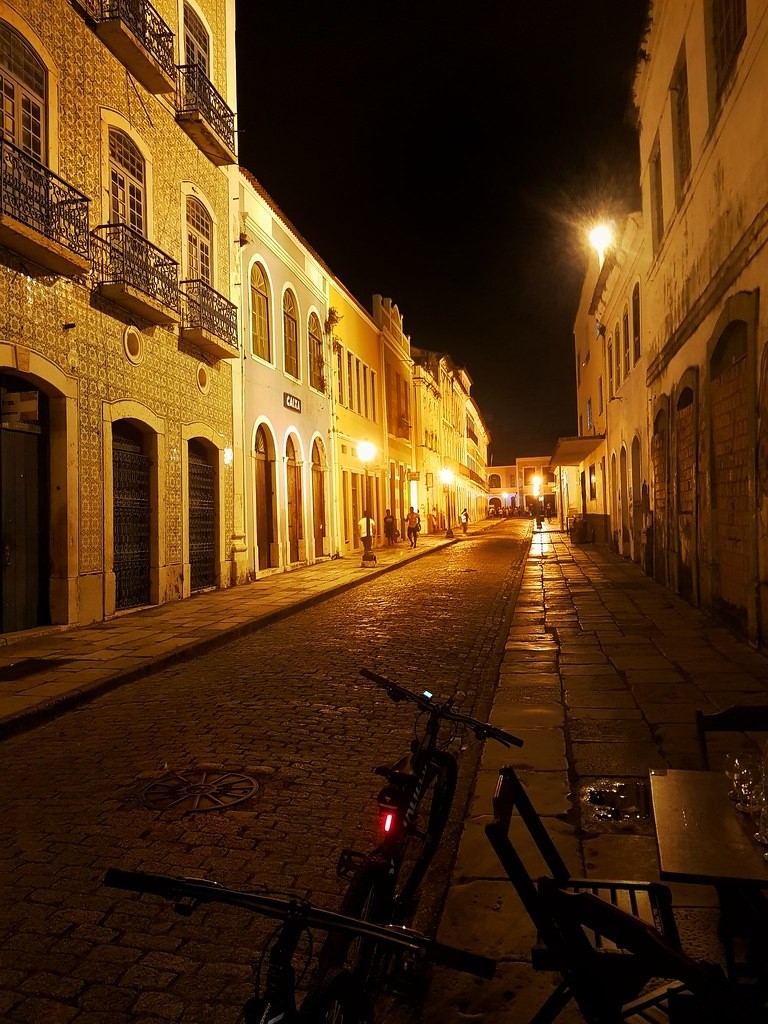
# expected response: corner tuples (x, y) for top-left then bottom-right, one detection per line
(461, 512), (466, 523)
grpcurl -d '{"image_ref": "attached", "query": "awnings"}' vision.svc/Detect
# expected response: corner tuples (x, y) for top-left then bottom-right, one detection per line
(549, 432), (605, 467)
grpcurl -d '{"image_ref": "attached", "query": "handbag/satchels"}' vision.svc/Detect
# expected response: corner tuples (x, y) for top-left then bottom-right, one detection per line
(415, 523), (421, 532)
(393, 529), (400, 543)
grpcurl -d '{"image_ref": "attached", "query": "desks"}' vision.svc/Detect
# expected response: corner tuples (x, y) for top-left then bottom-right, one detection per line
(649, 768), (768, 991)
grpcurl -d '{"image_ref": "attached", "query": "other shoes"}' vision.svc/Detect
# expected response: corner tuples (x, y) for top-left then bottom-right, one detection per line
(463, 532), (467, 534)
(411, 542), (413, 547)
(414, 545), (416, 548)
(389, 546), (391, 548)
(392, 544), (394, 547)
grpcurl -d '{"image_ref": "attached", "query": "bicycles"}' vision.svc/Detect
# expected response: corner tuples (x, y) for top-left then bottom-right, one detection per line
(317, 666), (526, 979)
(103, 867), (500, 1024)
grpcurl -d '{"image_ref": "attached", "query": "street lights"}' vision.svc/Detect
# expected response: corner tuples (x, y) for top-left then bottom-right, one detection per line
(504, 493), (507, 508)
(360, 444), (375, 561)
(533, 477), (542, 529)
(442, 471), (454, 538)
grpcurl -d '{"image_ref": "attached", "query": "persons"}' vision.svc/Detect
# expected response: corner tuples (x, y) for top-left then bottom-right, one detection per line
(384, 509), (394, 547)
(358, 511), (376, 551)
(461, 508), (469, 534)
(494, 502), (551, 518)
(405, 507), (420, 547)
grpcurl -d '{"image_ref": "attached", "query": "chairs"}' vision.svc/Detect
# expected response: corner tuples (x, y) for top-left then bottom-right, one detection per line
(696, 705), (768, 770)
(485, 765), (683, 1024)
(538, 875), (768, 1024)
